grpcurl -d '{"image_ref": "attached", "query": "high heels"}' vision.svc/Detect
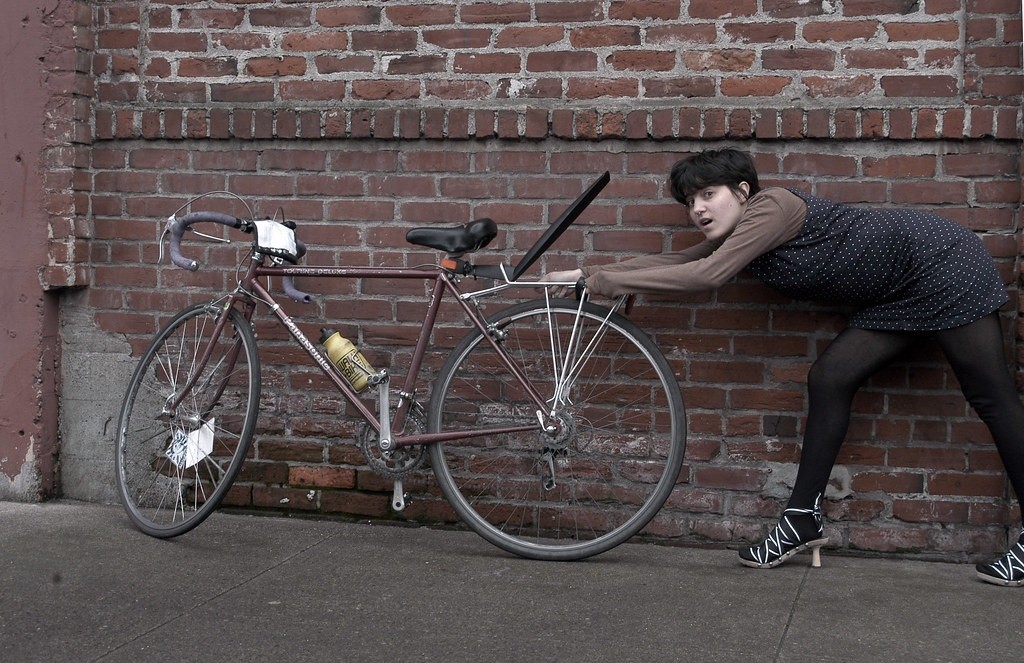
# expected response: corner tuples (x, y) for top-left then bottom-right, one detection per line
(974, 525), (1024, 586)
(737, 492), (829, 568)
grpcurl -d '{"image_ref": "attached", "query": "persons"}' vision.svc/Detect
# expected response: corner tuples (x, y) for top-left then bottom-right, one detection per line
(534, 150), (1024, 587)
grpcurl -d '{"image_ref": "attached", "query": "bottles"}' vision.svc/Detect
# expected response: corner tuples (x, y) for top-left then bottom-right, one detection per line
(318, 326), (376, 392)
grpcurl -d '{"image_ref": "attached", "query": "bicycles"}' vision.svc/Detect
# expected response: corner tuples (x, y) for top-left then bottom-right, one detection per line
(112, 162), (688, 560)
